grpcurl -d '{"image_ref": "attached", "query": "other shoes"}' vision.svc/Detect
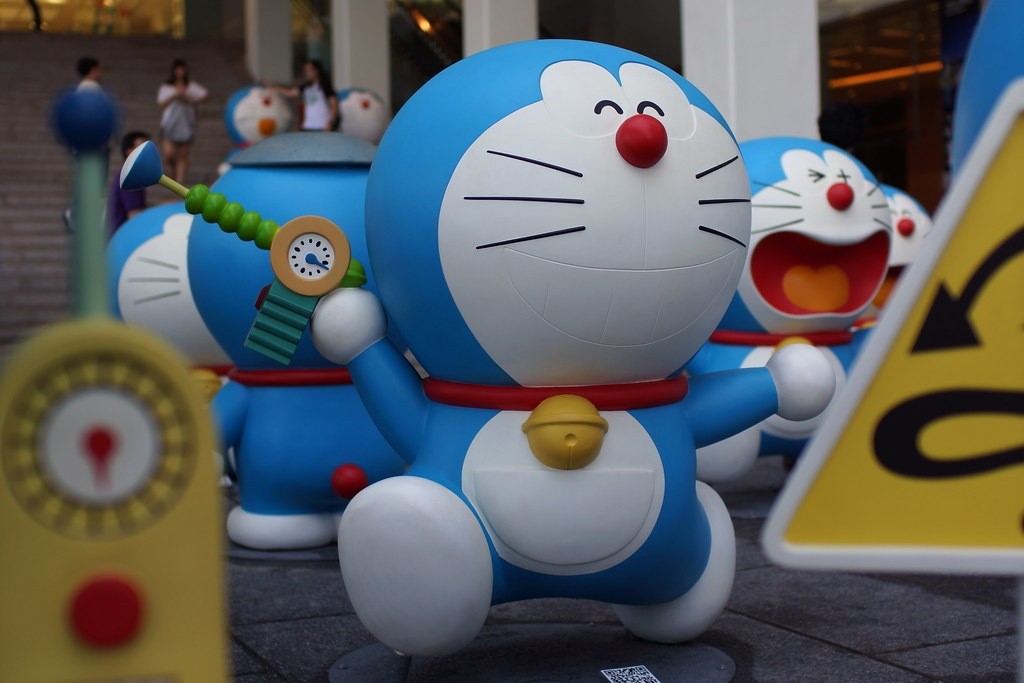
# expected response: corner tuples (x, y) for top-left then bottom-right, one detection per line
(64, 208), (74, 232)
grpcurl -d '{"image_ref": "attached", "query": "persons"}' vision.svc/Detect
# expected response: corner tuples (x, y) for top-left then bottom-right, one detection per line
(64, 56), (103, 229)
(106, 132), (154, 239)
(158, 59), (210, 185)
(264, 60), (342, 132)
